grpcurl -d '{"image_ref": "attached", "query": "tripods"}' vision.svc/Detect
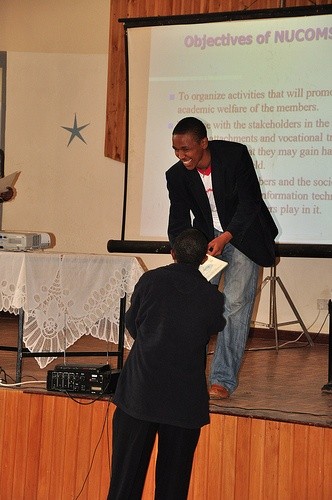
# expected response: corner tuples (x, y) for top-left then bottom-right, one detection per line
(254, 266), (314, 354)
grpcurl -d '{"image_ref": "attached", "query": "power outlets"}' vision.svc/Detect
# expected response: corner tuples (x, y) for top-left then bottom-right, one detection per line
(316, 299), (328, 310)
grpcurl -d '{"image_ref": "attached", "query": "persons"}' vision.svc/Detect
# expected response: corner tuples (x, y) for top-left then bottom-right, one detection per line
(0, 148), (15, 206)
(165, 116), (279, 400)
(105, 225), (226, 500)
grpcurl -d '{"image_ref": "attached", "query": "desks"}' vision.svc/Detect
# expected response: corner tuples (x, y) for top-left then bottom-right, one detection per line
(0, 249), (149, 388)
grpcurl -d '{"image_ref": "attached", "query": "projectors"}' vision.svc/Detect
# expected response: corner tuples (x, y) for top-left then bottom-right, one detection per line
(1, 229), (51, 251)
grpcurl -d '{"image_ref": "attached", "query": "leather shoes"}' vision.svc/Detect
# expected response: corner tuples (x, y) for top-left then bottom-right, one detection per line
(208, 384), (229, 401)
